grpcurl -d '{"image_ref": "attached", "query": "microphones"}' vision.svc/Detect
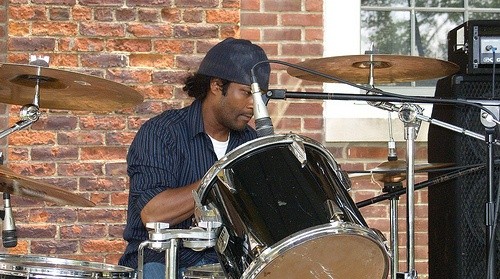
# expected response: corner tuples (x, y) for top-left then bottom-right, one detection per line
(250, 68), (274, 138)
(2, 192), (17, 248)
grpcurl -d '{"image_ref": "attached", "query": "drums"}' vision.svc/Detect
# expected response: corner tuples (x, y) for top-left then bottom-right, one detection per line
(0, 253), (137, 279)
(190, 130), (392, 279)
(182, 262), (228, 279)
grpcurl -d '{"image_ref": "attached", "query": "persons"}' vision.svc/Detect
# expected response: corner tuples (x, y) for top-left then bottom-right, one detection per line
(118, 37), (270, 279)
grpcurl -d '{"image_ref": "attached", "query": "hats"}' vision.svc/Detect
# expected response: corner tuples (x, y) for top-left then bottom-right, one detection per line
(197, 37), (271, 92)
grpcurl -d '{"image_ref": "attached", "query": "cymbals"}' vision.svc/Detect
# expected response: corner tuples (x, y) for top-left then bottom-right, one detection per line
(0, 62), (144, 112)
(287, 53), (461, 85)
(0, 164), (97, 208)
(347, 161), (458, 182)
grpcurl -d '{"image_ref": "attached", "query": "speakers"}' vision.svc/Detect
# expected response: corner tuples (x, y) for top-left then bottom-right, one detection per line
(426, 73), (500, 279)
